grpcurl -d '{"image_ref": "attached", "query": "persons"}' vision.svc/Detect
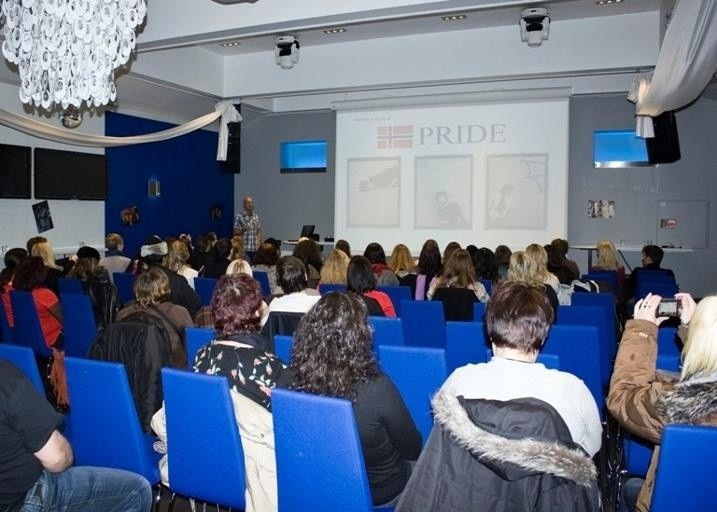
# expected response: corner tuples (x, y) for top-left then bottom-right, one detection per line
(411, 239), (681, 333)
(37, 204), (50, 233)
(433, 276), (606, 509)
(275, 287), (424, 512)
(358, 161), (399, 192)
(0, 231), (424, 435)
(487, 184), (515, 224)
(603, 288), (717, 511)
(435, 190), (468, 227)
(230, 196), (264, 259)
(0, 356), (155, 511)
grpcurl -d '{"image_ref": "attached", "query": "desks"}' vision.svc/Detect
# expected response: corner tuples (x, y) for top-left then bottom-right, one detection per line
(571, 243), (692, 277)
(283, 239), (333, 251)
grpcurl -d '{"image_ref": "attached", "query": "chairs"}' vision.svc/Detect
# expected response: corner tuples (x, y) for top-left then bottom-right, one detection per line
(1, 259), (716, 511)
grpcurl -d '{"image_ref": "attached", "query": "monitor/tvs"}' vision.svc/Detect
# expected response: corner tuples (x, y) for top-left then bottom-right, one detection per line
(0, 144), (31, 199)
(34, 147), (109, 200)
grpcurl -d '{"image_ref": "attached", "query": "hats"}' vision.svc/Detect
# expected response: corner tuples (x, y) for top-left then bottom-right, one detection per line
(77, 246), (100, 258)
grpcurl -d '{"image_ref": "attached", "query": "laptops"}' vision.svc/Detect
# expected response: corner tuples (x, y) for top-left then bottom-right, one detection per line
(288, 225), (315, 242)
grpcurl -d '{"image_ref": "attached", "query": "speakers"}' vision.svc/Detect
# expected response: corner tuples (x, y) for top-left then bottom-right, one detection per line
(219, 140), (240, 175)
(219, 103), (240, 139)
(645, 111), (681, 164)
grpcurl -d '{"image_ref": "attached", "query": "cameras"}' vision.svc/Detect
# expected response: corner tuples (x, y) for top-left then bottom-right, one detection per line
(656, 298), (682, 316)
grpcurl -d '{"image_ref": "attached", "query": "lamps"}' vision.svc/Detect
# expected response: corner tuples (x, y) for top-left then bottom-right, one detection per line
(1, 0), (150, 130)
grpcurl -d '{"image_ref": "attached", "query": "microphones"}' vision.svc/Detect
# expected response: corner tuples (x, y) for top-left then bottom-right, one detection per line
(249, 207), (253, 217)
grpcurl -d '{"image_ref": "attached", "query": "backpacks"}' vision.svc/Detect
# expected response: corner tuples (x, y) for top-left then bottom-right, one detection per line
(89, 282), (121, 326)
(570, 279), (624, 340)
